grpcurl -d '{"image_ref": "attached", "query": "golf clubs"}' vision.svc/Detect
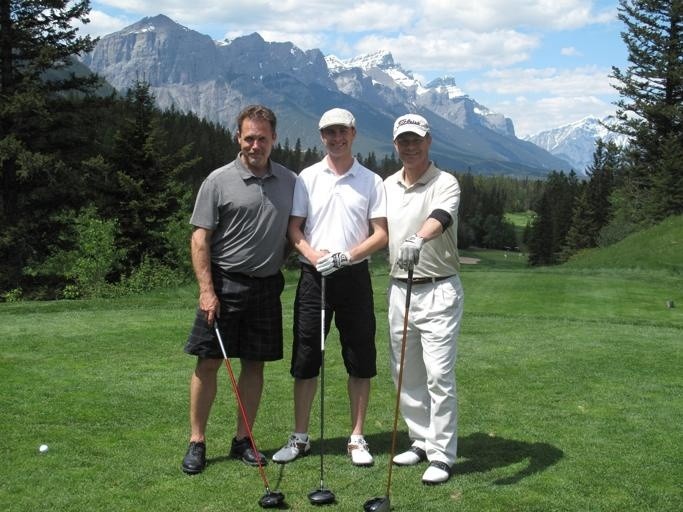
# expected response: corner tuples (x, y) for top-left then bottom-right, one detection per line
(308, 276), (335, 505)
(364, 271), (413, 512)
(214, 320), (284, 508)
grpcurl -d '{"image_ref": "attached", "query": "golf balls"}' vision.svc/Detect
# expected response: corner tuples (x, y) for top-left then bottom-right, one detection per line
(39, 444), (49, 454)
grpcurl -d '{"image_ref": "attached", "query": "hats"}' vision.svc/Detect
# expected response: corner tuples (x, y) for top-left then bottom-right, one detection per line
(318, 106), (356, 131)
(392, 113), (430, 140)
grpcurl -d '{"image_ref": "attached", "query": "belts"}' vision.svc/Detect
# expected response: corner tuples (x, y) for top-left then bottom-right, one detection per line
(398, 276), (455, 285)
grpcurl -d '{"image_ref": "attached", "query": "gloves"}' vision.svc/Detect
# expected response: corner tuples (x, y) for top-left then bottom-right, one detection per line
(395, 233), (424, 272)
(315, 250), (351, 278)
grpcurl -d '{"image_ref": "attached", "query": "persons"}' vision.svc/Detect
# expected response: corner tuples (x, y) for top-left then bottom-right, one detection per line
(271, 107), (389, 465)
(378, 114), (461, 485)
(180, 104), (299, 475)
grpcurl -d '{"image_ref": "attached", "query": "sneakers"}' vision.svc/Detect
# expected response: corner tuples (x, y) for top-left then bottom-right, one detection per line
(229, 435), (269, 467)
(347, 434), (374, 467)
(182, 438), (208, 474)
(272, 434), (312, 463)
(392, 446), (426, 466)
(422, 460), (452, 484)
